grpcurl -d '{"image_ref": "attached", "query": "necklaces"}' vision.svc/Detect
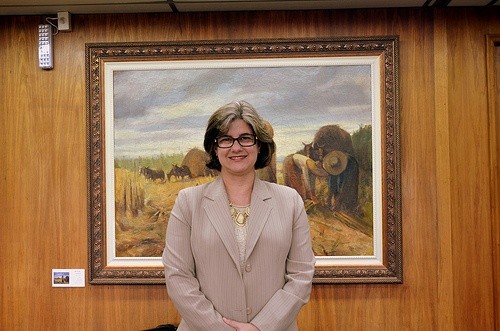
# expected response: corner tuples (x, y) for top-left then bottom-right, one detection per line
(228, 199), (250, 227)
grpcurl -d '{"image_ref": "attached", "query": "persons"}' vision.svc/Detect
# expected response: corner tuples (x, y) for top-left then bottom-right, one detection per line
(160, 99), (317, 331)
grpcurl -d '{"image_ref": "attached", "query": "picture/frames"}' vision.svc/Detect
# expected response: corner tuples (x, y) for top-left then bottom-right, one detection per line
(84, 34), (403, 284)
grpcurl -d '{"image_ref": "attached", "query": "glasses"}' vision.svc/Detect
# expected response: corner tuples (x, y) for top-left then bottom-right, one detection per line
(215, 134), (258, 148)
